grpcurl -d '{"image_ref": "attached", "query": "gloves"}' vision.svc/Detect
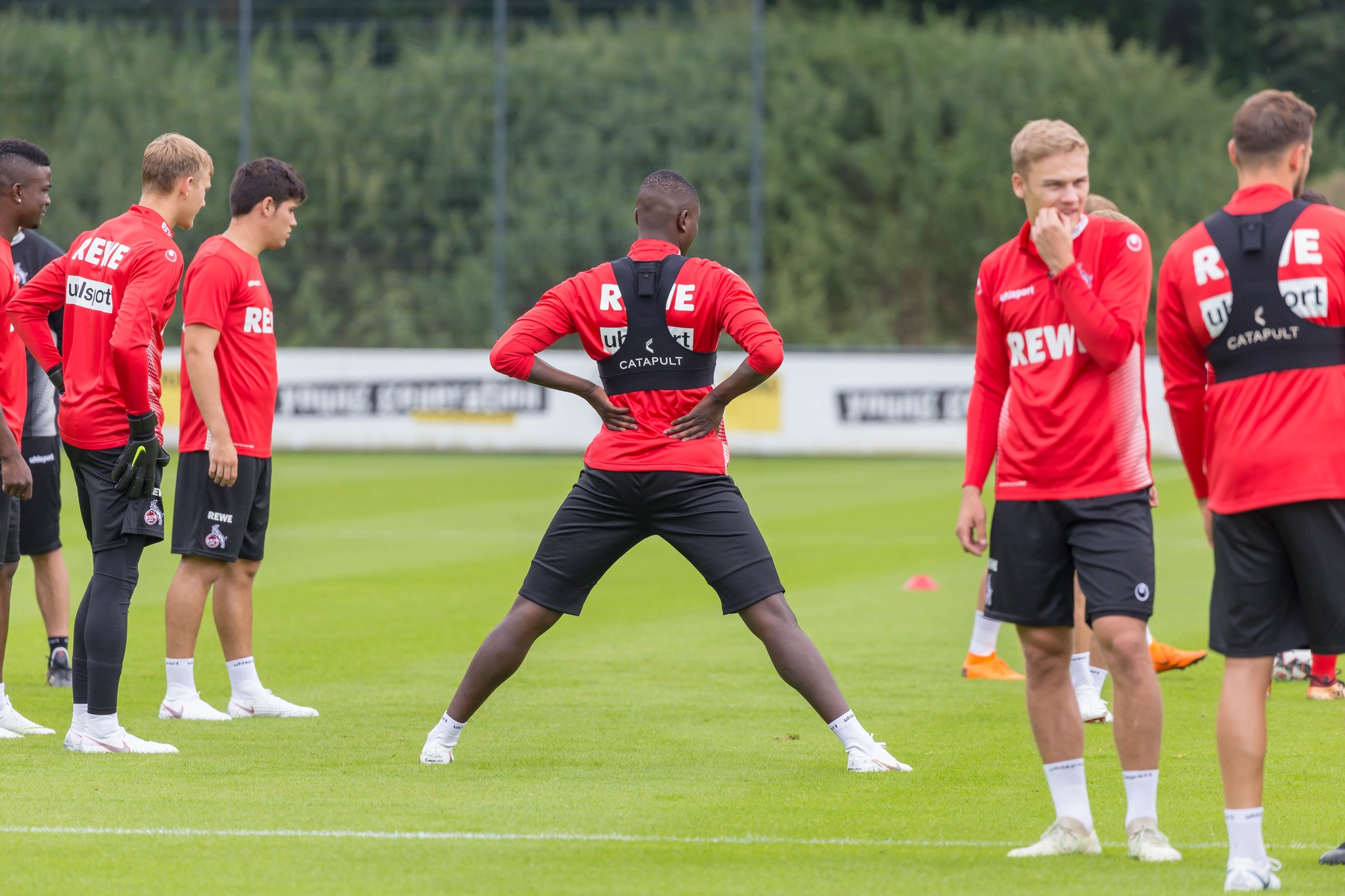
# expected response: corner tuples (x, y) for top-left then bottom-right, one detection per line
(47, 369), (66, 396)
(109, 412), (171, 501)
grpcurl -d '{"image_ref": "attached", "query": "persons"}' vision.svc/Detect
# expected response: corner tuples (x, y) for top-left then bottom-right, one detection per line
(959, 117), (1182, 866)
(157, 157), (323, 722)
(416, 170), (912, 776)
(958, 389), (1345, 723)
(0, 137), (54, 743)
(10, 226), (74, 691)
(1151, 86), (1345, 894)
(4, 132), (216, 763)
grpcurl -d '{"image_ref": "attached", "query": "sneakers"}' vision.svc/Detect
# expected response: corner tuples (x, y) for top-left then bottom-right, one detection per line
(1126, 817), (1182, 862)
(843, 733), (913, 772)
(63, 729), (83, 751)
(1225, 856), (1282, 892)
(962, 652), (1026, 680)
(79, 726), (179, 754)
(46, 646), (73, 687)
(0, 728), (24, 738)
(0, 694), (56, 735)
(1074, 684), (1108, 723)
(420, 730), (460, 765)
(1146, 637), (1208, 673)
(1307, 680), (1345, 701)
(159, 690), (232, 721)
(1092, 699), (1114, 723)
(1008, 816), (1101, 860)
(227, 688), (319, 719)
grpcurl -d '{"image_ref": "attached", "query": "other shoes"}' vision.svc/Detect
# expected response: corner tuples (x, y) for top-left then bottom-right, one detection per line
(1318, 843), (1345, 864)
